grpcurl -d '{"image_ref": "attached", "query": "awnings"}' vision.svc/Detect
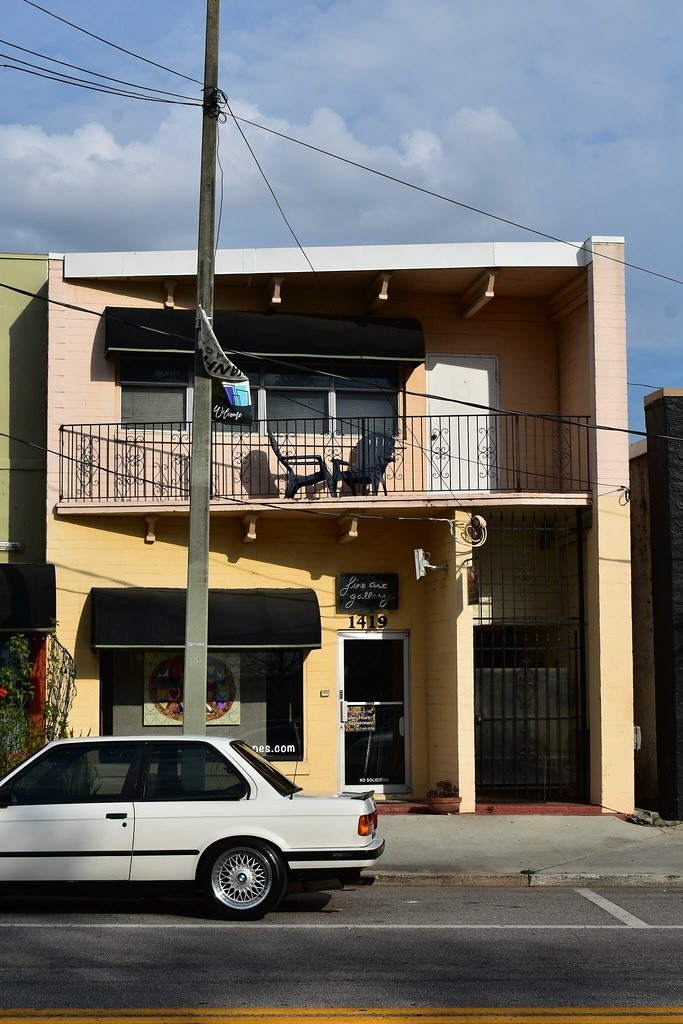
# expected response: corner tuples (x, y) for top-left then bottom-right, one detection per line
(91, 586), (322, 649)
(104, 306), (427, 363)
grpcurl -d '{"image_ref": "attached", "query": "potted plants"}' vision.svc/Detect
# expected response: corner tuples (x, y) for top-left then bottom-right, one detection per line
(424, 781), (461, 813)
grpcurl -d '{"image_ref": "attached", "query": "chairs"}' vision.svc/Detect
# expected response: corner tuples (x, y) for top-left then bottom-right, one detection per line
(332, 432), (394, 497)
(146, 771), (184, 797)
(267, 427), (333, 499)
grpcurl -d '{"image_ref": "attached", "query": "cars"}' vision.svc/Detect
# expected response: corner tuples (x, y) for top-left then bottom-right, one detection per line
(0, 736), (385, 922)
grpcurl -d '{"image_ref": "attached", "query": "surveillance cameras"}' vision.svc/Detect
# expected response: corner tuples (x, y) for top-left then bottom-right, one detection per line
(414, 549), (427, 581)
